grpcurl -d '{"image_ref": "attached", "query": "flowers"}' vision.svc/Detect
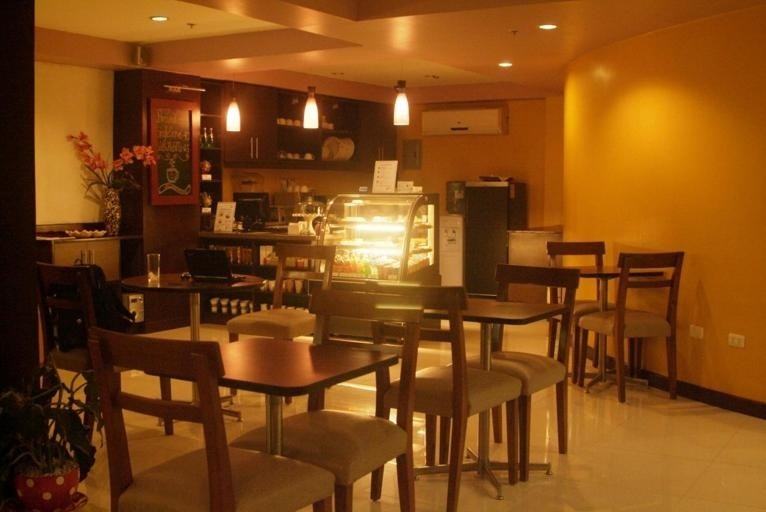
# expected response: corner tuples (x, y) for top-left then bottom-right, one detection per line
(66, 128), (158, 194)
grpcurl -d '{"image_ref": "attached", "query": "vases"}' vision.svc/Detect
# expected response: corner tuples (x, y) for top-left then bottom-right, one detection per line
(102, 188), (120, 236)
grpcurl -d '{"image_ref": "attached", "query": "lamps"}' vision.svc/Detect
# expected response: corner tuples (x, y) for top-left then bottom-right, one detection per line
(393, 60), (411, 126)
(225, 73), (241, 131)
(301, 67), (321, 130)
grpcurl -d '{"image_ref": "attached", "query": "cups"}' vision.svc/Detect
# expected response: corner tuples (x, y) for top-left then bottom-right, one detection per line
(278, 118), (300, 127)
(146, 253), (161, 284)
(286, 185), (309, 193)
(210, 297), (308, 315)
(321, 121), (334, 130)
(297, 259), (326, 272)
(267, 279), (305, 294)
(279, 151), (314, 161)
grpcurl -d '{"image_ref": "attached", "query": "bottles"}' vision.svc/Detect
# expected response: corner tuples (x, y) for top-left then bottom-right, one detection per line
(201, 127), (215, 145)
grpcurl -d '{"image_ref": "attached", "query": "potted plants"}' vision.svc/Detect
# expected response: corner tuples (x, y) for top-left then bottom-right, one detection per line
(0, 351), (113, 511)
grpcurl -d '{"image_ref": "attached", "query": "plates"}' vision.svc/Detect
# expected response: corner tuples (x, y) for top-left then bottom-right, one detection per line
(65, 229), (107, 238)
(322, 137), (354, 161)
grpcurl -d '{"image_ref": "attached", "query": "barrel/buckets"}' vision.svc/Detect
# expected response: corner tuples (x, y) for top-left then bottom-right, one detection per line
(445, 180), (465, 214)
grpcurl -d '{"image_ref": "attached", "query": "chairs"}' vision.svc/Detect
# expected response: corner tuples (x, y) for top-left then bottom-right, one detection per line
(37, 242), (685, 512)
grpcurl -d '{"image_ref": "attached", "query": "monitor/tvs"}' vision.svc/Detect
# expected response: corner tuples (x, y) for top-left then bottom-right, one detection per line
(232, 192), (269, 230)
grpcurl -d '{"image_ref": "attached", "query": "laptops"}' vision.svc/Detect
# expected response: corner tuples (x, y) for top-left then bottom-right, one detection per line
(184, 248), (244, 282)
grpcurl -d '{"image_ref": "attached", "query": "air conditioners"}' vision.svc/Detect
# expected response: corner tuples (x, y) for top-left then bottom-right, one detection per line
(421, 108), (509, 136)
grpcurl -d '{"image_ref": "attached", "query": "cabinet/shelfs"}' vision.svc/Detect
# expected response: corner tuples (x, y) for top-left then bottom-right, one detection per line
(198, 80), (223, 230)
(317, 192), (440, 282)
(359, 99), (399, 172)
(223, 82), (278, 163)
(278, 88), (361, 170)
